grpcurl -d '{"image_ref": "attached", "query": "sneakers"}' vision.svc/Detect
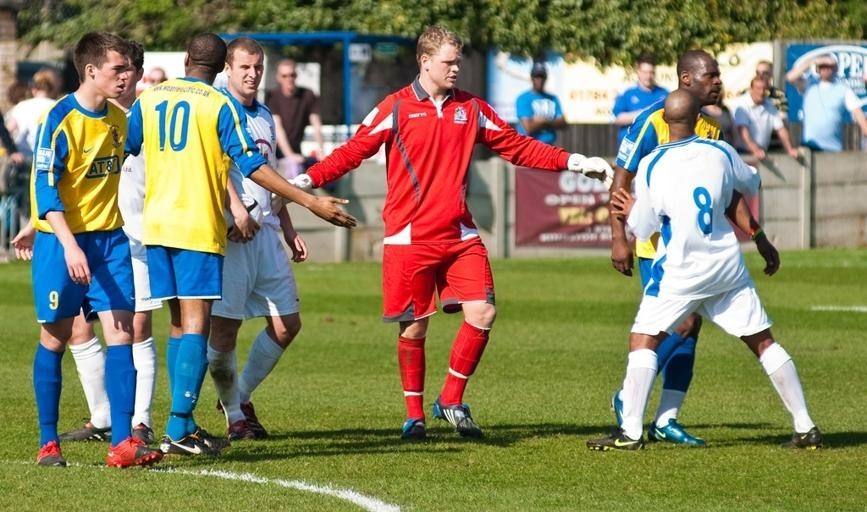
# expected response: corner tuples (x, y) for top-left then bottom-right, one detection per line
(587, 428), (645, 451)
(216, 398), (269, 440)
(401, 418), (426, 440)
(648, 419), (706, 447)
(58, 418), (112, 442)
(782, 426), (823, 450)
(106, 423), (231, 467)
(433, 397), (482, 440)
(37, 440), (66, 467)
(611, 388), (623, 428)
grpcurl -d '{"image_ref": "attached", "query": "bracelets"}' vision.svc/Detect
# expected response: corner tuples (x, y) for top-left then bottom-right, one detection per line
(751, 226), (764, 242)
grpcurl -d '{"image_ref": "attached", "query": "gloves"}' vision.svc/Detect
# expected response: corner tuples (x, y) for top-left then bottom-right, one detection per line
(271, 173), (313, 216)
(567, 152), (615, 183)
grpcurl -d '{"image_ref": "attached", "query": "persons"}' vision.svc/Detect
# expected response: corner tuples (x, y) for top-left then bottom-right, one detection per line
(612, 59), (669, 148)
(270, 27), (614, 442)
(585, 86), (823, 452)
(785, 54), (867, 152)
(0, 32), (356, 470)
(737, 59), (788, 149)
(731, 78), (804, 162)
(608, 49), (782, 445)
(516, 64), (566, 145)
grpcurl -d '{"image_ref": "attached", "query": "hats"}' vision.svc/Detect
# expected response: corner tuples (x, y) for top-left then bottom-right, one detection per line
(531, 63), (545, 77)
